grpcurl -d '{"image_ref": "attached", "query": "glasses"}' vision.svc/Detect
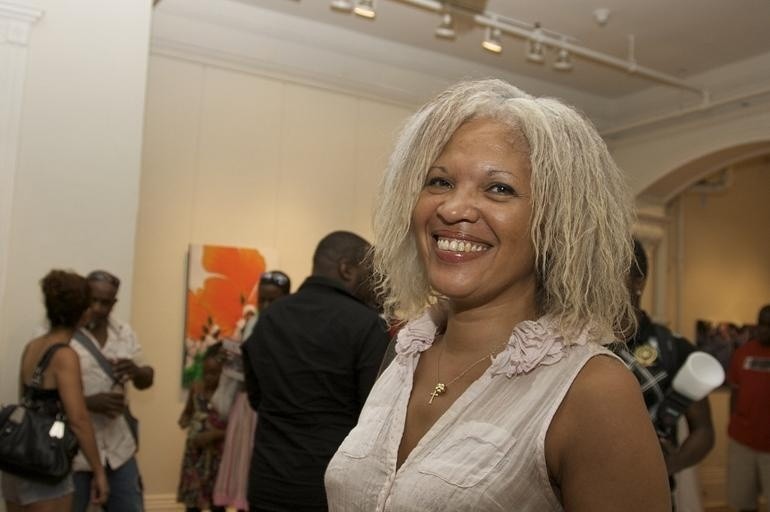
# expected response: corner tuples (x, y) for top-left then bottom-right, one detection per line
(258, 271), (289, 291)
(86, 271), (120, 289)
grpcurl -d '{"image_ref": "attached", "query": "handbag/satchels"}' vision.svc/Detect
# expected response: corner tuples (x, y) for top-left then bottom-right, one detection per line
(199, 315), (257, 422)
(0, 399), (79, 485)
(123, 405), (137, 452)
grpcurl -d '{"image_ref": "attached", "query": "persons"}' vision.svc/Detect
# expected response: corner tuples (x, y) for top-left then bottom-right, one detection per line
(212, 271), (293, 512)
(238, 228), (393, 512)
(175, 349), (229, 512)
(695, 305), (769, 512)
(69, 270), (160, 512)
(321, 76), (673, 512)
(1, 266), (112, 512)
(603, 235), (715, 512)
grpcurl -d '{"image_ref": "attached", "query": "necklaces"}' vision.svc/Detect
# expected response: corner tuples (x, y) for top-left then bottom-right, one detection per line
(429, 343), (506, 406)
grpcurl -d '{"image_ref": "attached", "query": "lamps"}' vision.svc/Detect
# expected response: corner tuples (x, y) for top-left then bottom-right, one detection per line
(330, 0), (578, 72)
